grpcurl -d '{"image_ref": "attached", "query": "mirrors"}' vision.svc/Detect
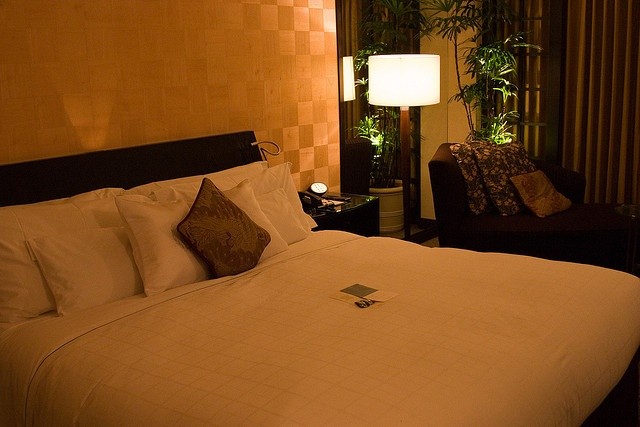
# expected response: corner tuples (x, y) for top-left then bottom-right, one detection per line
(335, 0), (420, 237)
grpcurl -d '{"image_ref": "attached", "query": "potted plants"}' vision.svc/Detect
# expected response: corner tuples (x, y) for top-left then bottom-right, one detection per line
(350, 1), (420, 233)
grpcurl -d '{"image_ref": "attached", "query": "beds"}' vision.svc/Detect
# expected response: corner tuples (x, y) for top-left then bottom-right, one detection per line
(0, 131), (640, 426)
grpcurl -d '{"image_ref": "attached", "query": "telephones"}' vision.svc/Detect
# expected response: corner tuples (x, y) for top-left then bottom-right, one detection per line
(297, 191), (336, 214)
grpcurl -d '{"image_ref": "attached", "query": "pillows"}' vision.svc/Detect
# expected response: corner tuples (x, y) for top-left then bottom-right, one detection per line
(115, 179), (289, 297)
(119, 161), (269, 195)
(256, 188), (309, 245)
(1, 187), (125, 333)
(177, 177), (271, 279)
(26, 227), (144, 317)
(472, 141), (538, 217)
(450, 140), (494, 216)
(148, 162), (318, 234)
(509, 170), (572, 217)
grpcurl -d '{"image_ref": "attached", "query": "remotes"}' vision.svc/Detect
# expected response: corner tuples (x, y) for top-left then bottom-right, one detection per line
(331, 194), (352, 202)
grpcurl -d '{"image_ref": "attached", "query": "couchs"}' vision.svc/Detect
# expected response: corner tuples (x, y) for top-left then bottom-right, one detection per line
(428, 144), (639, 277)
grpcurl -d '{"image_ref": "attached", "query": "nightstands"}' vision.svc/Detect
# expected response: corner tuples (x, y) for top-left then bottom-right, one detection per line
(299, 192), (380, 236)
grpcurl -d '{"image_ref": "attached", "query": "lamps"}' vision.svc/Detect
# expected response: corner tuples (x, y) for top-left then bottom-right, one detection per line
(368, 54), (440, 244)
(338, 56), (356, 103)
